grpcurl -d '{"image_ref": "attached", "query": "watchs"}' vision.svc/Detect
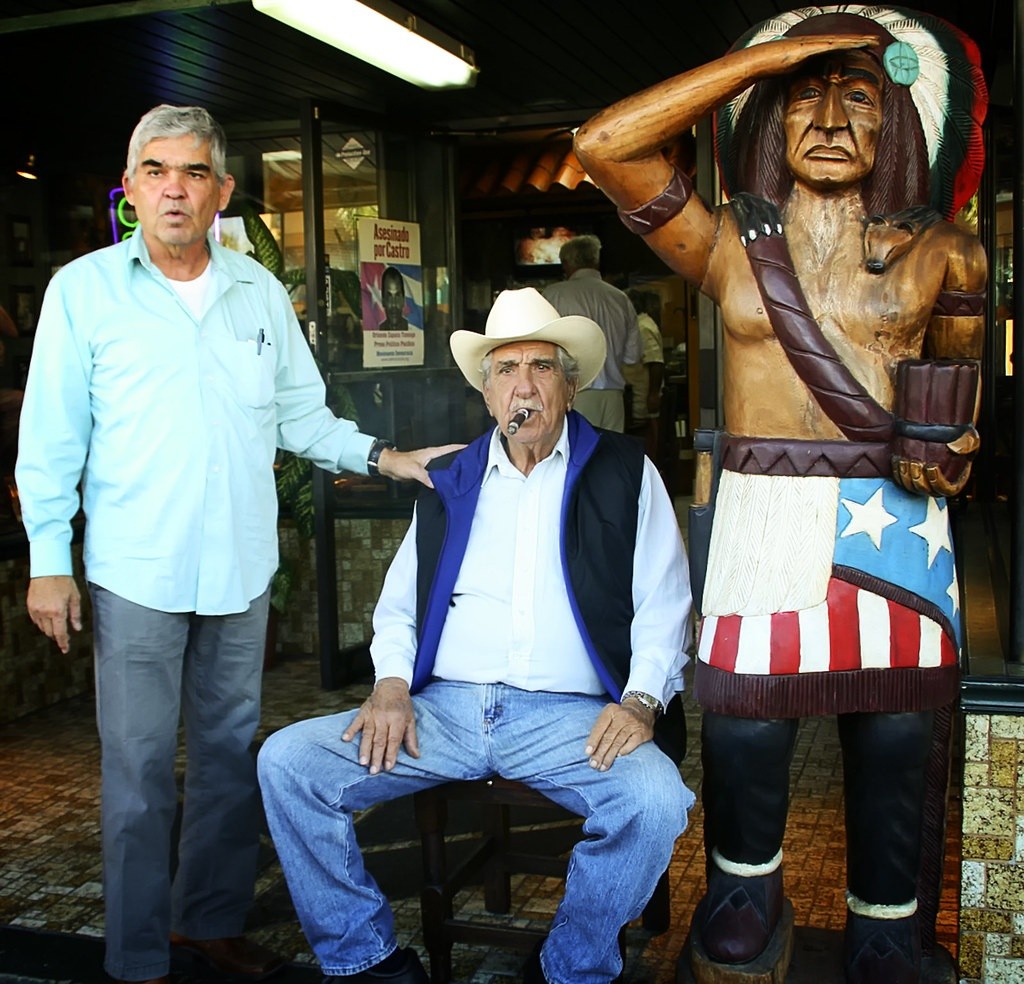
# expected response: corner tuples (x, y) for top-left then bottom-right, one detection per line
(367, 438), (400, 484)
(623, 690), (663, 720)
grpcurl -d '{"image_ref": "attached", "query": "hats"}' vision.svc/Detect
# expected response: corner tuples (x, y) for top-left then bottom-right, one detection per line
(450, 288), (606, 394)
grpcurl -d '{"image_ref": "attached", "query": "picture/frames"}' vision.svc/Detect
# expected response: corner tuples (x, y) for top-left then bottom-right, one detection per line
(8, 284), (36, 337)
(5, 213), (35, 268)
(13, 356), (30, 391)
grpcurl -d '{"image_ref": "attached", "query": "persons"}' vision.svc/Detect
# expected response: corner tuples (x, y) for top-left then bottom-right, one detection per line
(539, 234), (645, 435)
(620, 288), (667, 461)
(17, 102), (471, 984)
(570, 25), (992, 983)
(377, 266), (422, 333)
(254, 286), (698, 984)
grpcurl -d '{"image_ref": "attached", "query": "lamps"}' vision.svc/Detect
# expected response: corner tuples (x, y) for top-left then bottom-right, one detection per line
(252, 0), (481, 92)
(16, 161), (38, 181)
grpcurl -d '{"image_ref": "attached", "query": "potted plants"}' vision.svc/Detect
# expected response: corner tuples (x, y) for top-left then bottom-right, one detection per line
(243, 201), (359, 668)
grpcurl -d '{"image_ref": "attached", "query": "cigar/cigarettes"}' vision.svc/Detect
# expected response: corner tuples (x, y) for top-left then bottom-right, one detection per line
(507, 408), (533, 435)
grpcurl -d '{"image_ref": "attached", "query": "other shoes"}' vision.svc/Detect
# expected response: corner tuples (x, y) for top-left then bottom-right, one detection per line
(321, 947), (431, 984)
(523, 936), (548, 984)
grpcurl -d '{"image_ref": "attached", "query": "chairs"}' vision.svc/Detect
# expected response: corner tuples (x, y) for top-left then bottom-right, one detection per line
(414, 451), (687, 984)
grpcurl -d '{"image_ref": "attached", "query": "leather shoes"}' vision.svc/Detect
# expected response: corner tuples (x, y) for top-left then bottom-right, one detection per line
(124, 977), (169, 984)
(169, 931), (288, 981)
